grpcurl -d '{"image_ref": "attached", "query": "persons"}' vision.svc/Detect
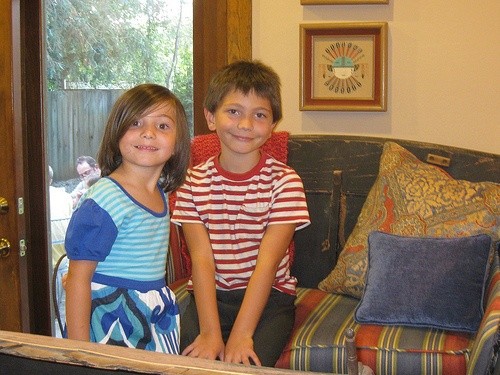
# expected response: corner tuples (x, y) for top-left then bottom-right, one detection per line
(48, 163), (73, 243)
(172, 59), (313, 366)
(57, 152), (102, 200)
(64, 84), (188, 357)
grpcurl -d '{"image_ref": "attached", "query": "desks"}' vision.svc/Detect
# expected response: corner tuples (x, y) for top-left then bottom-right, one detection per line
(0, 330), (345, 375)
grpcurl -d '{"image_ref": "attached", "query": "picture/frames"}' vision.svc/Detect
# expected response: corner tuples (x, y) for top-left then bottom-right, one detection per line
(299, 0), (388, 5)
(299, 22), (388, 112)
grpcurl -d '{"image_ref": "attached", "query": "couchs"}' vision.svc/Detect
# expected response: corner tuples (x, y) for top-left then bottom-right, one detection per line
(167, 134), (500, 375)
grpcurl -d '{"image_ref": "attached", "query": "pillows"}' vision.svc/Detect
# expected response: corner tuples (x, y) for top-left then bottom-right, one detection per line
(352, 231), (492, 332)
(317, 143), (500, 299)
